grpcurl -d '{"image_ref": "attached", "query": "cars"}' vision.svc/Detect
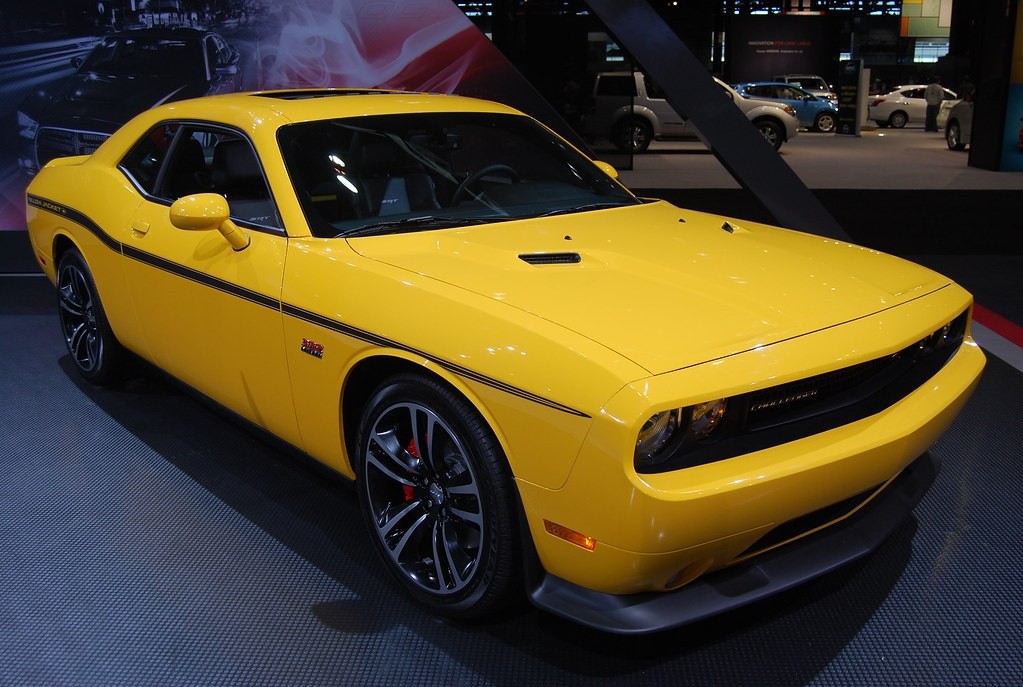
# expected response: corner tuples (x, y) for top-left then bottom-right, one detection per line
(945, 88), (974, 150)
(15, 25), (242, 193)
(869, 84), (957, 128)
(25, 87), (987, 638)
(737, 82), (839, 133)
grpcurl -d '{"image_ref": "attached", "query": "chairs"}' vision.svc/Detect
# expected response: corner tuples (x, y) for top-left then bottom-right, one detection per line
(208, 139), (280, 229)
(337, 123), (443, 221)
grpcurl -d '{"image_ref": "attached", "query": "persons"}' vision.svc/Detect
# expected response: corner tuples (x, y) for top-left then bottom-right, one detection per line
(923, 75), (944, 133)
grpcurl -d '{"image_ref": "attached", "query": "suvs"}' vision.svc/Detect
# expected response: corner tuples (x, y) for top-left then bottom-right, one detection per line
(590, 72), (800, 154)
(771, 74), (839, 104)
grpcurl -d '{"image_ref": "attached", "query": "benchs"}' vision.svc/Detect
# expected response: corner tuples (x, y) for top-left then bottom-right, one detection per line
(160, 134), (331, 200)
